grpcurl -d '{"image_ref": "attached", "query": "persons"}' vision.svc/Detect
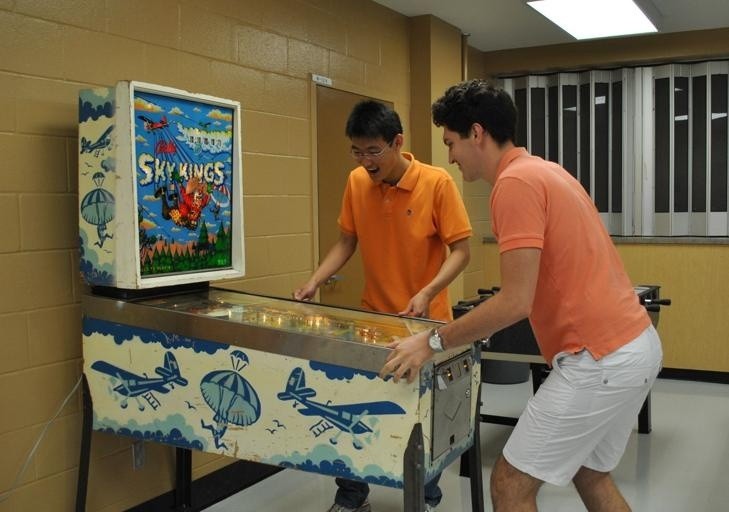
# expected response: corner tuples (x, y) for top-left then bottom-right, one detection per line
(293, 100), (474, 512)
(379, 78), (664, 511)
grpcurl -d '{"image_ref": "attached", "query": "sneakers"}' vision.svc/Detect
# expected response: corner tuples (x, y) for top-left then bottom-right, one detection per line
(327, 499), (371, 512)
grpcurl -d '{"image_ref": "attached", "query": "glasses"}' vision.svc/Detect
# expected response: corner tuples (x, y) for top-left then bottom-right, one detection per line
(351, 140), (392, 159)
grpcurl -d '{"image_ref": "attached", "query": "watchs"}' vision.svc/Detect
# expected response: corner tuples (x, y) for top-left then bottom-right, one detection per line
(429, 326), (446, 353)
